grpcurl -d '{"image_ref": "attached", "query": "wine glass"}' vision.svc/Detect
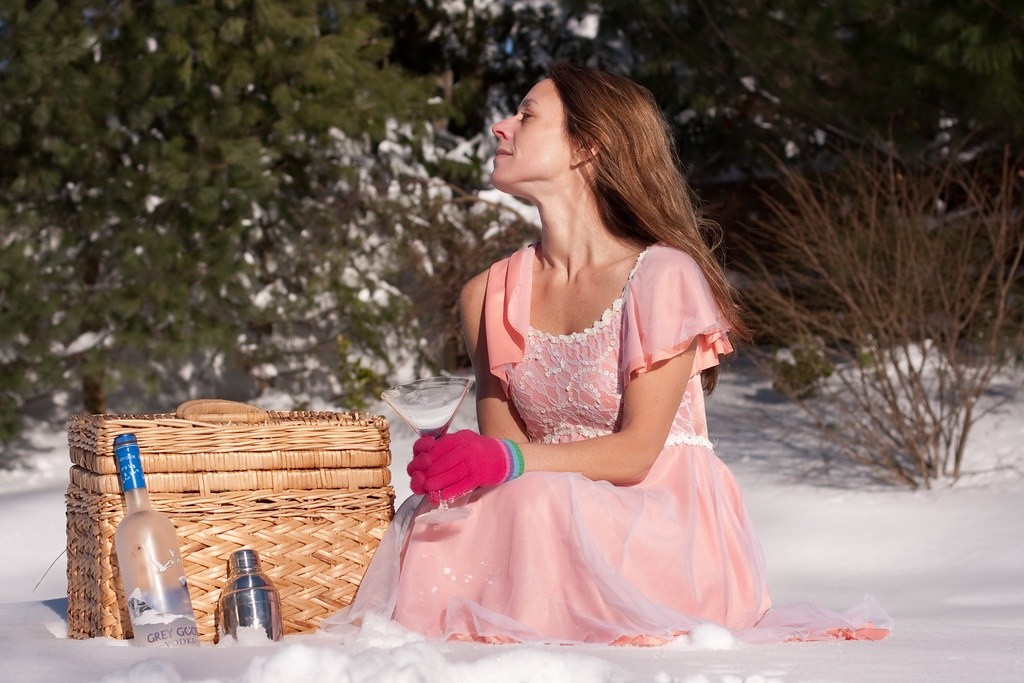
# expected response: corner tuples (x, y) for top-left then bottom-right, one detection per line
(381, 378), (474, 522)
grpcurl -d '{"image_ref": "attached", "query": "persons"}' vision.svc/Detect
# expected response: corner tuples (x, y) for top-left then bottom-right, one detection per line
(321, 64), (770, 648)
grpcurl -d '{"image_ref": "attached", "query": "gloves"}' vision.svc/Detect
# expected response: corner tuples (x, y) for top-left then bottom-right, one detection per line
(406, 429), (524, 507)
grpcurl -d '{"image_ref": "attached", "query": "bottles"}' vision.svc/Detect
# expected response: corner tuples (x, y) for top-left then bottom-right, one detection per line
(217, 549), (283, 643)
(113, 433), (200, 647)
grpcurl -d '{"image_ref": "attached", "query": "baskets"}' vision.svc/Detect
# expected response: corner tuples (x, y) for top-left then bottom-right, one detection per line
(63, 399), (398, 645)
